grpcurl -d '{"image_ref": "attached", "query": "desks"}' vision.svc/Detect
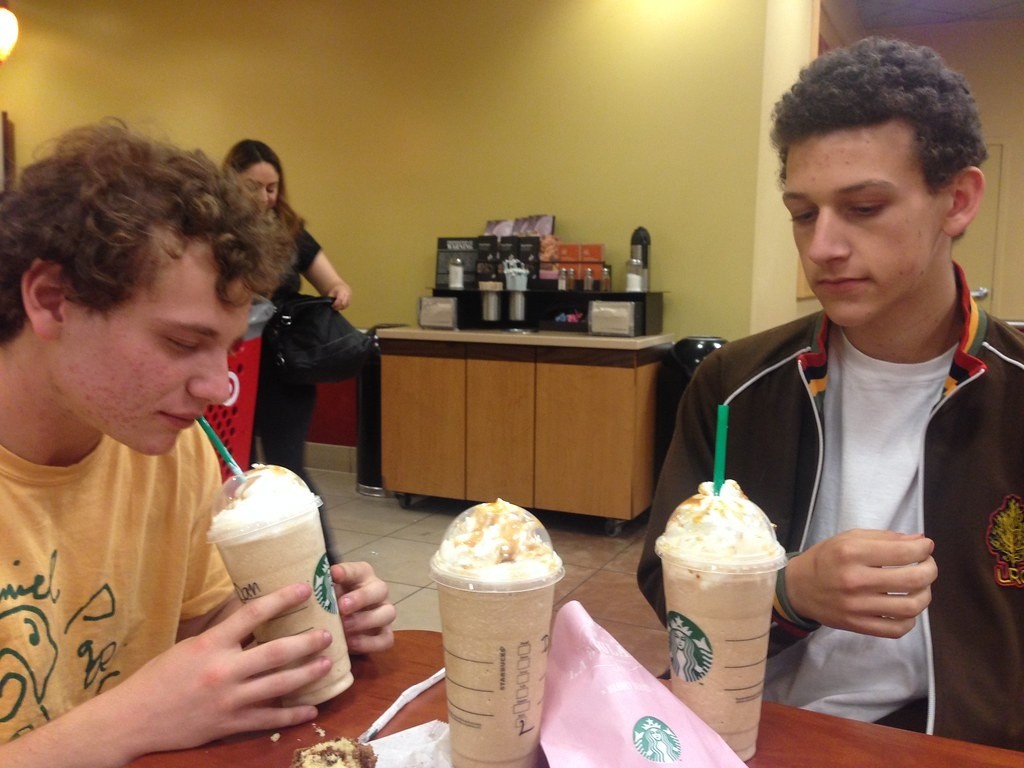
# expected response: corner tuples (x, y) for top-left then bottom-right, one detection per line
(122, 628), (1024, 767)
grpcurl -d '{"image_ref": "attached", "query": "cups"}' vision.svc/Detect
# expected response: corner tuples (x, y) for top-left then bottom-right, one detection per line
(654, 492), (789, 762)
(205, 465), (354, 709)
(429, 502), (566, 768)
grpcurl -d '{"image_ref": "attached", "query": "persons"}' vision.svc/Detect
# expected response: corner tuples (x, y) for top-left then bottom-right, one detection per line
(1, 116), (398, 768)
(638, 35), (1024, 748)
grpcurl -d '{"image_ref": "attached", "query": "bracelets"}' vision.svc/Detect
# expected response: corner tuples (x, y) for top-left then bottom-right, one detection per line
(776, 550), (823, 632)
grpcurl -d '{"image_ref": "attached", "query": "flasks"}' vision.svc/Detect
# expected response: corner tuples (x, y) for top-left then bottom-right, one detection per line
(631, 226), (651, 292)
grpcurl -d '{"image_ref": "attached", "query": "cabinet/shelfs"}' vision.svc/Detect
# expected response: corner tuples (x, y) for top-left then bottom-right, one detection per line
(377, 324), (674, 536)
(427, 284), (669, 335)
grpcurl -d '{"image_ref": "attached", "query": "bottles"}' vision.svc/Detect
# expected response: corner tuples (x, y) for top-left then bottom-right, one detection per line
(448, 256), (464, 290)
(600, 267), (611, 292)
(583, 268), (593, 291)
(558, 268), (567, 290)
(624, 258), (643, 293)
(567, 267), (576, 290)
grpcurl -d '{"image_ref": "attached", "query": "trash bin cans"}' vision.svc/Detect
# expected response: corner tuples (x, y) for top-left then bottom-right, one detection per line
(354, 321), (410, 498)
(653, 334), (730, 488)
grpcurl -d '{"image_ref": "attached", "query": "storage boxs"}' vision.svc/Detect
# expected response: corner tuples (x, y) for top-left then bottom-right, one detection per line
(436, 233), (605, 290)
